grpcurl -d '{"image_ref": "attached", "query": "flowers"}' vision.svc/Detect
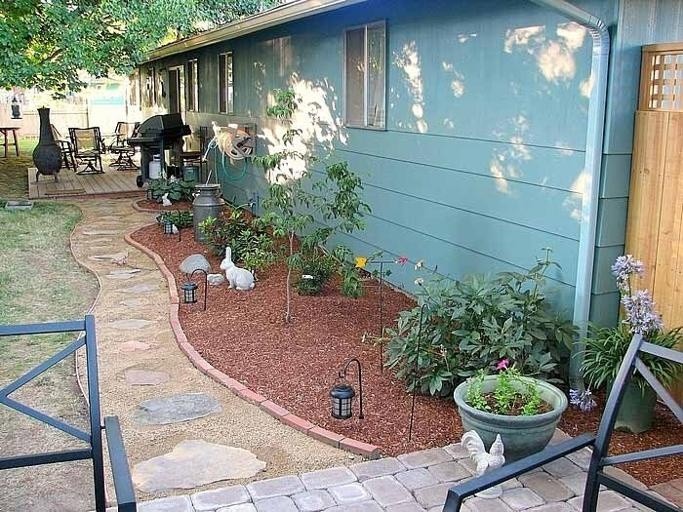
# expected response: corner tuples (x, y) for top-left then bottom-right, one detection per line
(569, 251), (683, 411)
(466, 356), (541, 415)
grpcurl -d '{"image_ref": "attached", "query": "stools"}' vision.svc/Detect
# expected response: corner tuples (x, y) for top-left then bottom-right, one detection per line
(0, 127), (21, 158)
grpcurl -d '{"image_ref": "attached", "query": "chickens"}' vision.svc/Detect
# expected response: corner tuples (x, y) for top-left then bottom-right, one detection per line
(460, 429), (506, 478)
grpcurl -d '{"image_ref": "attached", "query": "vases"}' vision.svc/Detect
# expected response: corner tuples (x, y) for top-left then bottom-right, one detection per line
(610, 377), (657, 434)
(454, 375), (568, 468)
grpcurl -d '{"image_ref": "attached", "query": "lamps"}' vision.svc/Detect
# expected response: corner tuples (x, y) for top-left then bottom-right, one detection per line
(181, 268), (208, 311)
(328, 359), (366, 420)
(164, 207), (181, 243)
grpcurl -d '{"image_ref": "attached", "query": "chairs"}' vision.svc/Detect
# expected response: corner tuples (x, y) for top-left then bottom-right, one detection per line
(68, 127), (106, 174)
(50, 123), (76, 173)
(174, 126), (208, 184)
(108, 121), (140, 171)
(0, 315), (138, 512)
(443, 332), (683, 512)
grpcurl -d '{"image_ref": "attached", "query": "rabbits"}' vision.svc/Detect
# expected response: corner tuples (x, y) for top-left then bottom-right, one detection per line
(207, 273), (225, 286)
(220, 246), (255, 290)
(162, 193), (173, 207)
